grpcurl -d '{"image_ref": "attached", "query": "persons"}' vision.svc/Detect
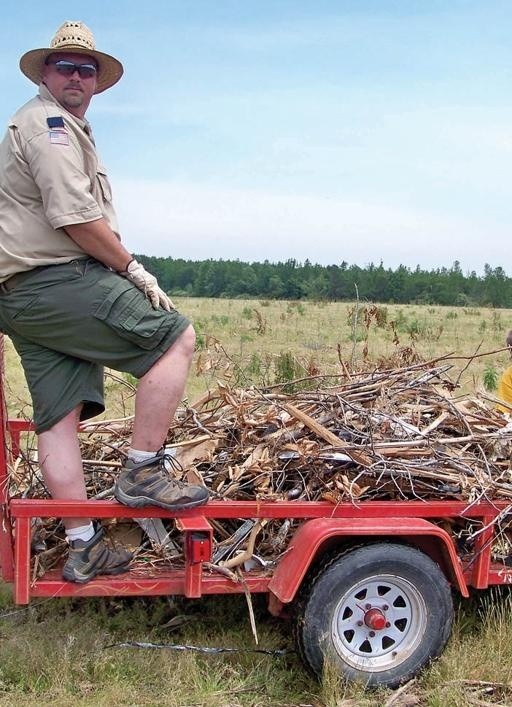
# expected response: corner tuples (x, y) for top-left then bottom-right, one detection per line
(0, 20), (210, 584)
(497, 330), (512, 415)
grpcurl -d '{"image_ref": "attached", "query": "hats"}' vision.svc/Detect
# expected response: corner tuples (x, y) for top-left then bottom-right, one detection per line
(18, 20), (124, 96)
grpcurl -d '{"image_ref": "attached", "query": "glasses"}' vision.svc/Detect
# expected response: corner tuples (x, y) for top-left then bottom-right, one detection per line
(46, 60), (98, 80)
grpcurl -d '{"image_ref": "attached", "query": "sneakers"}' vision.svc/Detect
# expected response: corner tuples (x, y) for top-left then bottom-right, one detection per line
(61, 523), (135, 585)
(114, 446), (211, 511)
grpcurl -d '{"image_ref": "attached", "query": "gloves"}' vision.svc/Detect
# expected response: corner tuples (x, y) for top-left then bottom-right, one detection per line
(118, 258), (177, 312)
